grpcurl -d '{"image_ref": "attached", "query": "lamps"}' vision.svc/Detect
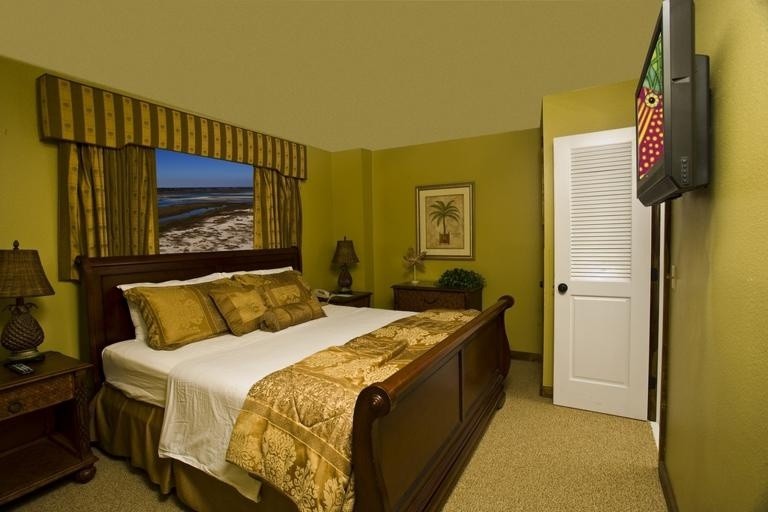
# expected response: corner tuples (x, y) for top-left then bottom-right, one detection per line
(0, 238), (55, 364)
(330, 233), (358, 295)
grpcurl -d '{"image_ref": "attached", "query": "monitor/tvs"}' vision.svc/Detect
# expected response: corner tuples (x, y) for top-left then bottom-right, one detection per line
(633, 0), (711, 207)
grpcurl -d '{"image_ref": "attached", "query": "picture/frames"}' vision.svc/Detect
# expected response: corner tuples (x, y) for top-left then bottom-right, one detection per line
(415, 180), (474, 262)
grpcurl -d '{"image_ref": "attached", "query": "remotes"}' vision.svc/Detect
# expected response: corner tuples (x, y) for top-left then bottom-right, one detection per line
(11, 362), (34, 374)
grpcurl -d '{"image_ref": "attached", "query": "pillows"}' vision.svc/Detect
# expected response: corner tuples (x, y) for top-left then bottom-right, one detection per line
(118, 264), (325, 352)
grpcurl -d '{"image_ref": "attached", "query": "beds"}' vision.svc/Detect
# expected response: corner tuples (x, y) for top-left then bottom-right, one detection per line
(75, 244), (514, 511)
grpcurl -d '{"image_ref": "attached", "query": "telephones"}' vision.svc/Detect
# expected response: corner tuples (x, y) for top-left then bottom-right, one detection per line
(313, 289), (329, 298)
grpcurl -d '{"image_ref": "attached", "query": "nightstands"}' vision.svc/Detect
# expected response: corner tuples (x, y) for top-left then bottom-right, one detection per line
(0, 349), (96, 512)
(317, 287), (373, 308)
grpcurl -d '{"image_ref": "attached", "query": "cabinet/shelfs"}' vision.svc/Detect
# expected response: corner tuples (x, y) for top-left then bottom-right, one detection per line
(390, 280), (484, 315)
